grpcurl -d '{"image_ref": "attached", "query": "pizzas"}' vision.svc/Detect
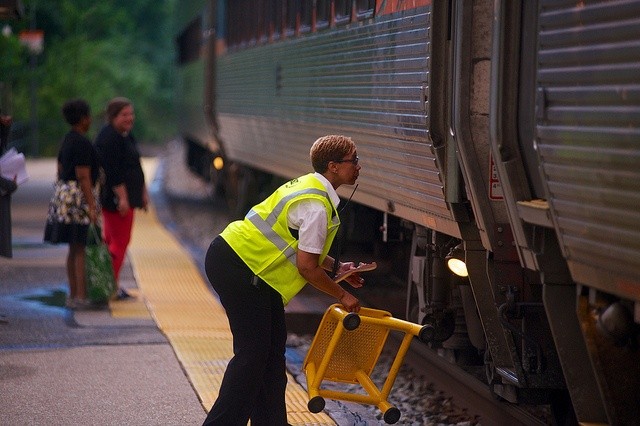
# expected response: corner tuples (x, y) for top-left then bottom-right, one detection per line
(331, 261), (378, 284)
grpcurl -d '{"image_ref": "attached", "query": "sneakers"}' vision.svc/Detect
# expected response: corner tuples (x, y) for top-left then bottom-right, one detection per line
(117, 287), (136, 301)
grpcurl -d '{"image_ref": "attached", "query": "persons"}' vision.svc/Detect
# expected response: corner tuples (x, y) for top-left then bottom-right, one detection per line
(93, 94), (149, 302)
(199, 130), (366, 425)
(43, 97), (100, 309)
(0, 110), (17, 259)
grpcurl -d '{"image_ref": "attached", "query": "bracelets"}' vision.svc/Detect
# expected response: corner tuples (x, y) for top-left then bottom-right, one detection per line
(331, 260), (340, 269)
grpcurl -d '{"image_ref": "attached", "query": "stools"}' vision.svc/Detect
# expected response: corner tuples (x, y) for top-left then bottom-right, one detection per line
(301, 304), (436, 424)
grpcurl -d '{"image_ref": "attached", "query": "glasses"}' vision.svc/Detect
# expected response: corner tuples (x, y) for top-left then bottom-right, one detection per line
(336, 155), (360, 165)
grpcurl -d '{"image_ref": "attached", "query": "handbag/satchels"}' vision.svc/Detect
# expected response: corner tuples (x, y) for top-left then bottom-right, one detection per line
(83, 220), (118, 302)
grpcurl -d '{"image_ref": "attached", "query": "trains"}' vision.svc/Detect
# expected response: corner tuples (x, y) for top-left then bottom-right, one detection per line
(166, 0), (640, 423)
